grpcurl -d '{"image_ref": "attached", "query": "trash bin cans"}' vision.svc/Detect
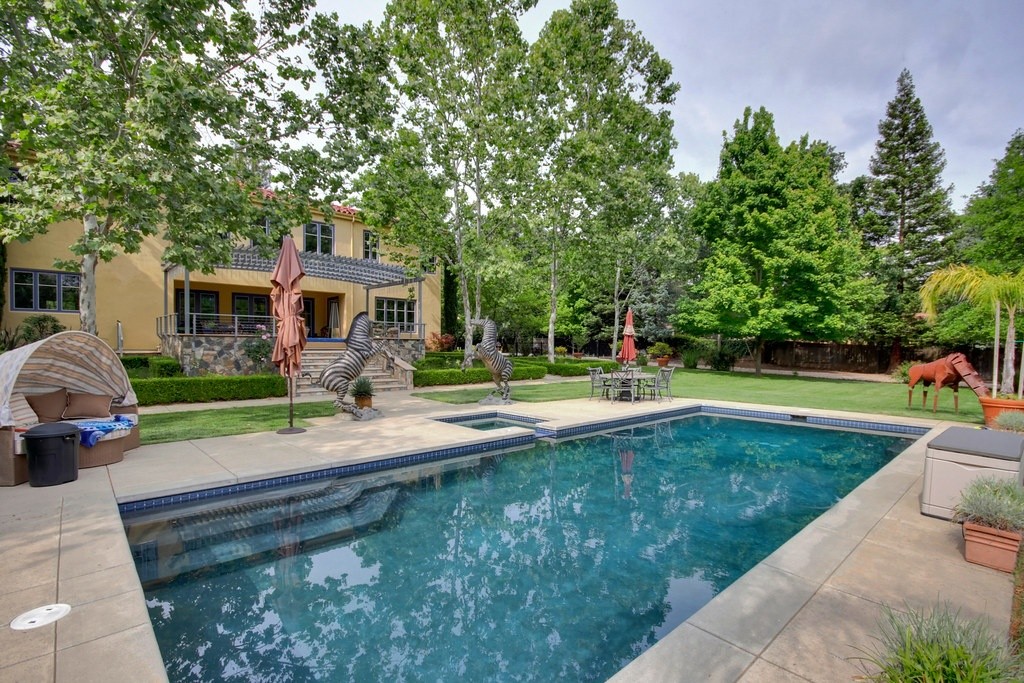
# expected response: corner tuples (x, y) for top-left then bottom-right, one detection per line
(19, 422), (85, 487)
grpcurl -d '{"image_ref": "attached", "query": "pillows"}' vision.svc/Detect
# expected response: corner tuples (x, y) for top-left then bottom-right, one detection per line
(63, 392), (113, 419)
(9, 392), (39, 428)
(26, 388), (69, 422)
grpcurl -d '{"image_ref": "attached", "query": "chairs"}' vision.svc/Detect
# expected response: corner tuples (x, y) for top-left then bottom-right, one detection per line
(587, 366), (675, 404)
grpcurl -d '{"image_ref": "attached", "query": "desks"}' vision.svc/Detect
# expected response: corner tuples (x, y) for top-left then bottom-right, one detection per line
(598, 373), (656, 400)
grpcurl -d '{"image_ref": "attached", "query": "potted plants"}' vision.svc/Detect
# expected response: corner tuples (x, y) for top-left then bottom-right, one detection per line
(348, 376), (376, 409)
(979, 392), (1024, 432)
(609, 339), (625, 365)
(645, 341), (675, 367)
(952, 472), (1024, 574)
(572, 335), (591, 358)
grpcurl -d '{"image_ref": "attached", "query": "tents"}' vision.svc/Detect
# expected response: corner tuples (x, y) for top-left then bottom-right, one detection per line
(0, 330), (138, 427)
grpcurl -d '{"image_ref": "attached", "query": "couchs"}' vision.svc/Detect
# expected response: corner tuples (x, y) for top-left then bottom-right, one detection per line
(0, 401), (140, 487)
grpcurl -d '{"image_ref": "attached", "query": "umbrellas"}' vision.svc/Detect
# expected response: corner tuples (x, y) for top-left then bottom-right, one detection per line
(618, 310), (640, 367)
(268, 233), (310, 428)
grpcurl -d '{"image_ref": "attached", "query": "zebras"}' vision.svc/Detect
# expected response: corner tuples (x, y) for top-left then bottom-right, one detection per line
(302, 310), (388, 418)
(462, 315), (513, 400)
